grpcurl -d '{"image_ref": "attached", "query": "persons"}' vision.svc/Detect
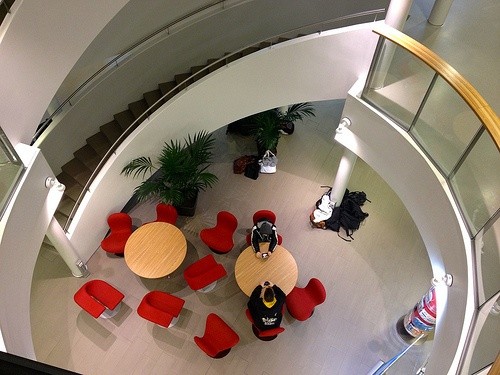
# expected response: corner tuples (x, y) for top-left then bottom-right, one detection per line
(247, 281), (286, 330)
(251, 219), (279, 259)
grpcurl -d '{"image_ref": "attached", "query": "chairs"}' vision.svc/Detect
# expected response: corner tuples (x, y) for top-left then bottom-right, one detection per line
(142, 204), (179, 227)
(73, 280), (125, 319)
(100, 212), (133, 254)
(200, 210), (238, 255)
(245, 303), (285, 337)
(286, 277), (326, 321)
(136, 288), (185, 329)
(194, 312), (240, 358)
(245, 209), (283, 246)
(183, 253), (227, 293)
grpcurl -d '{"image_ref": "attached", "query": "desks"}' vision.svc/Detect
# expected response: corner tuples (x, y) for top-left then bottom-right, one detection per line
(124, 222), (187, 279)
(235, 242), (298, 302)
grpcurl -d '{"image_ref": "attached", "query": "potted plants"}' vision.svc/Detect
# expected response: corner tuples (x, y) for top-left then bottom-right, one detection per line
(254, 101), (317, 159)
(120, 129), (221, 217)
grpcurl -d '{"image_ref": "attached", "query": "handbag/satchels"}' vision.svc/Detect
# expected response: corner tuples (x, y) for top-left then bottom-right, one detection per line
(263, 150), (279, 167)
(258, 159), (276, 173)
(233, 156), (251, 174)
(310, 188), (368, 237)
(244, 162), (261, 179)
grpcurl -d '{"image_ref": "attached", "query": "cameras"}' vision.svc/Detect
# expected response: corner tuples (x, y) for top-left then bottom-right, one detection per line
(262, 253), (269, 258)
(264, 282), (269, 286)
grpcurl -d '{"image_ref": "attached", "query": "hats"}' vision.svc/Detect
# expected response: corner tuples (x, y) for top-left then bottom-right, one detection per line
(260, 223), (271, 234)
